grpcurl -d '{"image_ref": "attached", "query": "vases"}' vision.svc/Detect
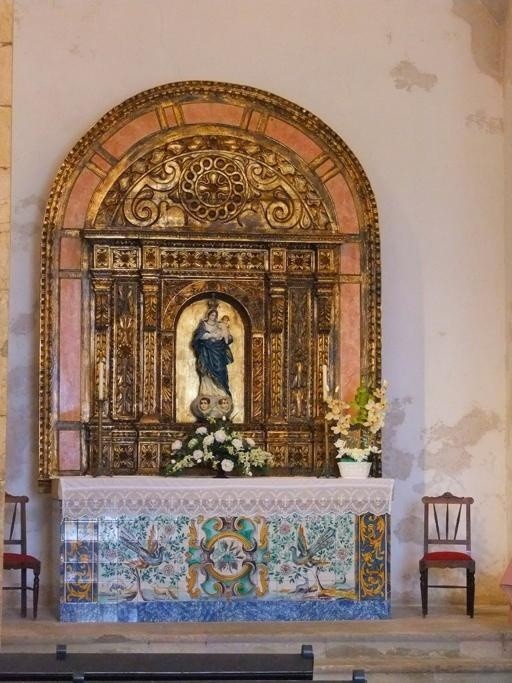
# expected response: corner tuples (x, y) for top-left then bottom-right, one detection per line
(337, 459), (373, 479)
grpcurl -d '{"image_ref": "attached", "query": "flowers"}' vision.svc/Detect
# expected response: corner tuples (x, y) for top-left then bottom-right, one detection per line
(163, 412), (281, 479)
(318, 374), (393, 463)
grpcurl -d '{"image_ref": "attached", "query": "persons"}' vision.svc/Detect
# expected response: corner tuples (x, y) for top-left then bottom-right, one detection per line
(191, 308), (233, 396)
(212, 316), (230, 344)
(219, 398), (229, 410)
(200, 398), (210, 410)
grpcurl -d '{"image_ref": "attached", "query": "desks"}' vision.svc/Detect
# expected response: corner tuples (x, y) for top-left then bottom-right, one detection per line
(48, 466), (396, 628)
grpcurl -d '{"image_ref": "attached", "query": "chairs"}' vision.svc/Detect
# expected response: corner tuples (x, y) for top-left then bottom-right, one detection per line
(0, 490), (41, 619)
(419, 487), (476, 619)
